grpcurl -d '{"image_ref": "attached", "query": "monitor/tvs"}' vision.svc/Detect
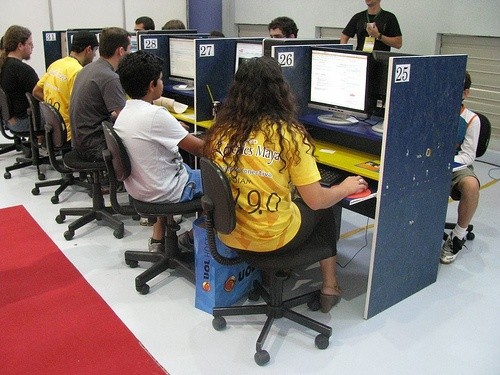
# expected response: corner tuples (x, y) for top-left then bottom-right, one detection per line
(234, 39), (263, 74)
(127, 33), (136, 53)
(168, 35), (203, 83)
(307, 46), (369, 118)
(60, 31), (68, 59)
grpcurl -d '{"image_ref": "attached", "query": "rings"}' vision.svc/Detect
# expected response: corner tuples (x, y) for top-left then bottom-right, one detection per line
(359, 178), (364, 184)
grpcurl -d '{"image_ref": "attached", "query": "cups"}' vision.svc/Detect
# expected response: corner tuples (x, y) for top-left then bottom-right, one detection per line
(367, 22), (374, 29)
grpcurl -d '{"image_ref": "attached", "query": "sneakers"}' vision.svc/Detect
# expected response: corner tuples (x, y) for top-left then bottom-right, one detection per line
(147, 230), (194, 256)
(440, 232), (468, 263)
(139, 213), (183, 226)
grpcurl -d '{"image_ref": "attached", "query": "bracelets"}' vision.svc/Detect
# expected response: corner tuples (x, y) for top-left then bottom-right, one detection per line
(377, 33), (382, 41)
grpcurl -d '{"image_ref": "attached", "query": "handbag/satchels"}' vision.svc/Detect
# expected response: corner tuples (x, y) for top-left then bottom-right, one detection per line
(191, 213), (264, 315)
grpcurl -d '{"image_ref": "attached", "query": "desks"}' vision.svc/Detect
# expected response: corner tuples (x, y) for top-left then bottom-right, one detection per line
(42, 26), (470, 322)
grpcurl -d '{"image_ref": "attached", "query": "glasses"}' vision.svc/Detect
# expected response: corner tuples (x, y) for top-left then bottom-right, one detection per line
(20, 40), (33, 47)
(269, 33), (288, 38)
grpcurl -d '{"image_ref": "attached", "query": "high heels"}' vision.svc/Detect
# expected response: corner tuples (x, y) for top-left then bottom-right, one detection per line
(319, 283), (343, 314)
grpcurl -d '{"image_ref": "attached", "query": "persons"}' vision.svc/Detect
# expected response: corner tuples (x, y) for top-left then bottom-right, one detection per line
(0, 25), (48, 153)
(133, 17), (156, 31)
(441, 70), (481, 264)
(161, 20), (186, 30)
(203, 57), (369, 322)
(268, 16), (300, 39)
(33, 30), (100, 149)
(340, 0), (402, 52)
(112, 47), (208, 260)
(70, 26), (133, 163)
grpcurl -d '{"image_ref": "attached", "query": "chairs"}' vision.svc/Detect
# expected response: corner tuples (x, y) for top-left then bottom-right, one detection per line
(443, 110), (493, 242)
(25, 92), (103, 205)
(0, 116), (42, 158)
(200, 157), (333, 366)
(0, 89), (64, 181)
(38, 100), (141, 241)
(102, 120), (203, 296)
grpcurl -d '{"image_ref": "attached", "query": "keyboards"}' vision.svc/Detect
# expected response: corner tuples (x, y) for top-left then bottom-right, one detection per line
(316, 166), (343, 187)
(191, 132), (205, 139)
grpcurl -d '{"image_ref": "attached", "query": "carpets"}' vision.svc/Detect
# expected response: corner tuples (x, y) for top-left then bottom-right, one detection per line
(0, 204), (172, 375)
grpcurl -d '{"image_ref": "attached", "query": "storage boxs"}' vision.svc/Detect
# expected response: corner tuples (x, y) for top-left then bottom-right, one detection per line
(191, 214), (264, 317)
